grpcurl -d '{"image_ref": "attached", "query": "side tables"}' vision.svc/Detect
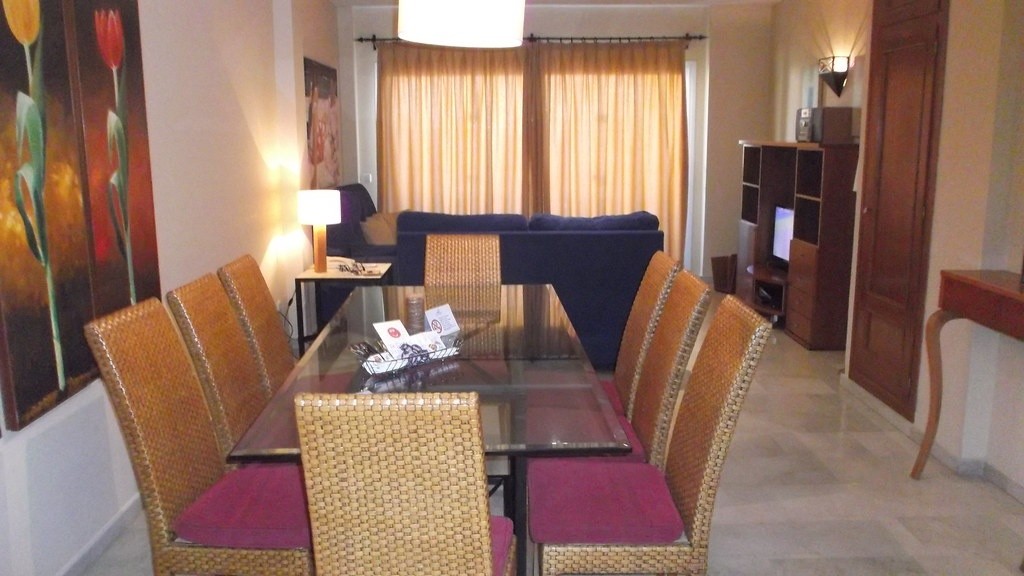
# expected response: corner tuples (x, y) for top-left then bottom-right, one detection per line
(294, 263), (395, 360)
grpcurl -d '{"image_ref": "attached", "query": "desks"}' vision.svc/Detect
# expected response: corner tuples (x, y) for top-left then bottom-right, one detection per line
(910, 269), (1024, 481)
(225, 284), (632, 576)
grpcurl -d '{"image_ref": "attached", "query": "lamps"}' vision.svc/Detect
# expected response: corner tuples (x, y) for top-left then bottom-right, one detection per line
(295, 189), (341, 273)
(399, 0), (527, 49)
(817, 55), (849, 98)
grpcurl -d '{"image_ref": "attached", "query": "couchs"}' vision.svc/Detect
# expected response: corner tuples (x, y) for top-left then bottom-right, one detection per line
(323, 183), (664, 371)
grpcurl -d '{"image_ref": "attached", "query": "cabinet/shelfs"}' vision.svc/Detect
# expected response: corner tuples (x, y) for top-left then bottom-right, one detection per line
(733, 141), (859, 351)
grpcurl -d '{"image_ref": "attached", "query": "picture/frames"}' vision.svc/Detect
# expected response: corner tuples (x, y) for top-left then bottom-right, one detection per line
(0, 0), (100, 432)
(69, 0), (162, 379)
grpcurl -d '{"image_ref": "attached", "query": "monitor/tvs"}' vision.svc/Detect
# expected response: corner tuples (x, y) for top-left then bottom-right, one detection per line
(770, 204), (794, 268)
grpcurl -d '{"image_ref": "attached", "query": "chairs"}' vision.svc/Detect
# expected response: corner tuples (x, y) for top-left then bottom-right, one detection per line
(83, 253), (773, 576)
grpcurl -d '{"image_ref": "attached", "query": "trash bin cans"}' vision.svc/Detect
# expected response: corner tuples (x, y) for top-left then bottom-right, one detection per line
(711, 254), (738, 295)
